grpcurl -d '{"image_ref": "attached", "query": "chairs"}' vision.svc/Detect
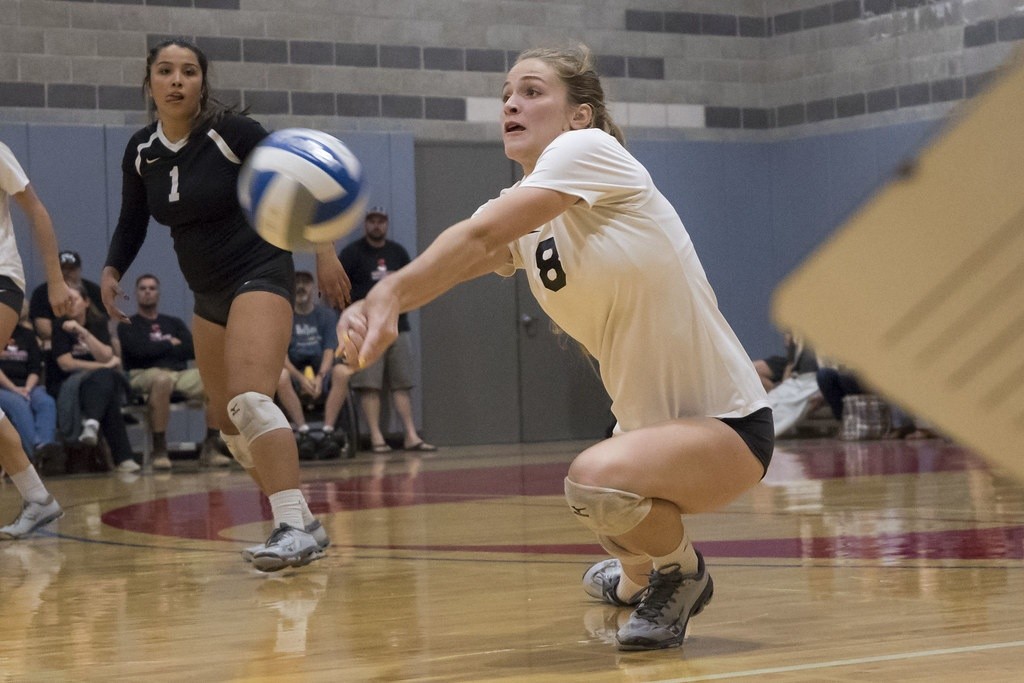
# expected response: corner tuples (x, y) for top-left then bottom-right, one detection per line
(116, 386), (359, 470)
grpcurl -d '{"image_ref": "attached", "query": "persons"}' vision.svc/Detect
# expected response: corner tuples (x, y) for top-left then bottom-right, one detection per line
(0, 296), (59, 466)
(336, 36), (776, 650)
(752, 330), (818, 392)
(100, 39), (352, 572)
(338, 203), (436, 453)
(0, 141), (76, 539)
(117, 274), (231, 469)
(46, 279), (139, 471)
(28, 249), (112, 398)
(275, 270), (352, 459)
(815, 359), (937, 439)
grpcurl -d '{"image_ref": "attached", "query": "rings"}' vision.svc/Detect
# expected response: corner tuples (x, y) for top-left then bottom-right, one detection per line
(335, 278), (345, 284)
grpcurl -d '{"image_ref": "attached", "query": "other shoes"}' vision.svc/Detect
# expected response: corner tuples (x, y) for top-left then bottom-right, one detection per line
(36, 441), (54, 454)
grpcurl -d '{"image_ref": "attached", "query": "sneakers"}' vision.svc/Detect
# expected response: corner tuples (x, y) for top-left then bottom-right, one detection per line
(240, 519), (330, 562)
(319, 433), (342, 459)
(298, 431), (317, 460)
(114, 459), (140, 472)
(581, 558), (641, 607)
(0, 493), (63, 540)
(252, 522), (326, 572)
(198, 446), (232, 467)
(78, 424), (98, 447)
(616, 548), (714, 651)
(153, 452), (172, 470)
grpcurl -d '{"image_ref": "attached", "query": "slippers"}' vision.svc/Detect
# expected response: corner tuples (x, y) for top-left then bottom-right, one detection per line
(404, 441), (438, 452)
(370, 443), (394, 454)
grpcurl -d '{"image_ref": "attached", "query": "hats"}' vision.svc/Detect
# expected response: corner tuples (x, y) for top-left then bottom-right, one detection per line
(366, 206), (388, 220)
(58, 250), (81, 270)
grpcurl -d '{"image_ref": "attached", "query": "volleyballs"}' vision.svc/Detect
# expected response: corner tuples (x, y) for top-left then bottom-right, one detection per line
(237, 127), (368, 252)
